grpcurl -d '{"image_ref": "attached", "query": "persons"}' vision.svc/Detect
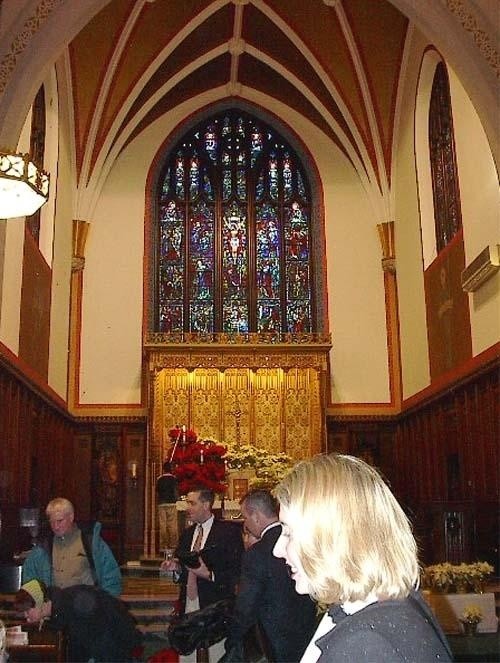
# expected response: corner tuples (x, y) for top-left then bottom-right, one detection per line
(13, 578), (144, 663)
(155, 460), (181, 505)
(21, 497), (123, 600)
(272, 449), (457, 663)
(160, 486), (246, 663)
(224, 491), (319, 663)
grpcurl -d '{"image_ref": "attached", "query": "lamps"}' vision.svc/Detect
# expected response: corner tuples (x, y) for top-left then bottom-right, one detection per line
(0, 149), (50, 220)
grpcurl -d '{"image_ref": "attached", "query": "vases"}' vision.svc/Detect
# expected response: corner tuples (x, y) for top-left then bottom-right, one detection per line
(457, 583), (471, 593)
(459, 618), (478, 636)
(442, 584), (451, 592)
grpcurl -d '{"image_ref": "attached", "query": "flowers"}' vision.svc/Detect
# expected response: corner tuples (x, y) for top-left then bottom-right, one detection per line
(168, 429), (292, 494)
(425, 561), (495, 588)
(463, 606), (484, 622)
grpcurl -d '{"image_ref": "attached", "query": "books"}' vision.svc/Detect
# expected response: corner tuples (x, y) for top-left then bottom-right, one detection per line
(3, 624), (31, 646)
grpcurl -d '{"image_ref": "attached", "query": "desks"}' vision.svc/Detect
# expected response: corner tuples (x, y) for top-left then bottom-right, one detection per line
(425, 593), (499, 635)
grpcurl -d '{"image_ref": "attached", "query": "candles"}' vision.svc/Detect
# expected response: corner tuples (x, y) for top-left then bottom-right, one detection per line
(132, 464), (136, 477)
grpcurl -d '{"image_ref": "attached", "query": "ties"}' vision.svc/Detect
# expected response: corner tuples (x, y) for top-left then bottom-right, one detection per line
(185, 526), (204, 600)
